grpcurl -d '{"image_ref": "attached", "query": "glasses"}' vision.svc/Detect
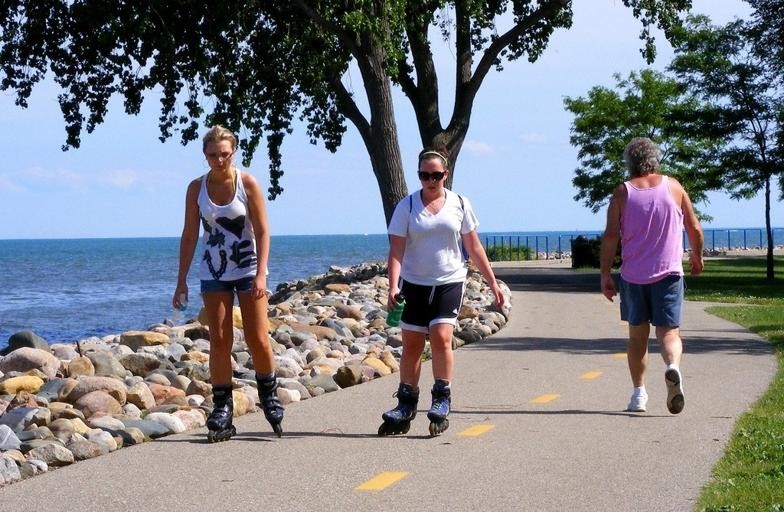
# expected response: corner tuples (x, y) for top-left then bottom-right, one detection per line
(418, 170), (446, 182)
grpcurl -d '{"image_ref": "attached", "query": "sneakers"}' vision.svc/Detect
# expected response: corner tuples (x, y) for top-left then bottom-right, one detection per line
(664, 365), (685, 414)
(627, 392), (648, 413)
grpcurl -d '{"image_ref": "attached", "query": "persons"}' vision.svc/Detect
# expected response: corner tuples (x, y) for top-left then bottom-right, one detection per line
(599, 136), (706, 413)
(380, 143), (504, 422)
(172, 123), (286, 430)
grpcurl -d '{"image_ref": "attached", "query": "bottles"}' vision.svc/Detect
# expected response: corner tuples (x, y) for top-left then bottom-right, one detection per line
(386, 293), (404, 327)
(172, 293), (187, 322)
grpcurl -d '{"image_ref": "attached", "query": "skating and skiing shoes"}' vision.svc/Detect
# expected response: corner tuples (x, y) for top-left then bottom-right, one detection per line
(254, 372), (284, 437)
(378, 382), (420, 437)
(205, 384), (236, 444)
(428, 378), (452, 436)
